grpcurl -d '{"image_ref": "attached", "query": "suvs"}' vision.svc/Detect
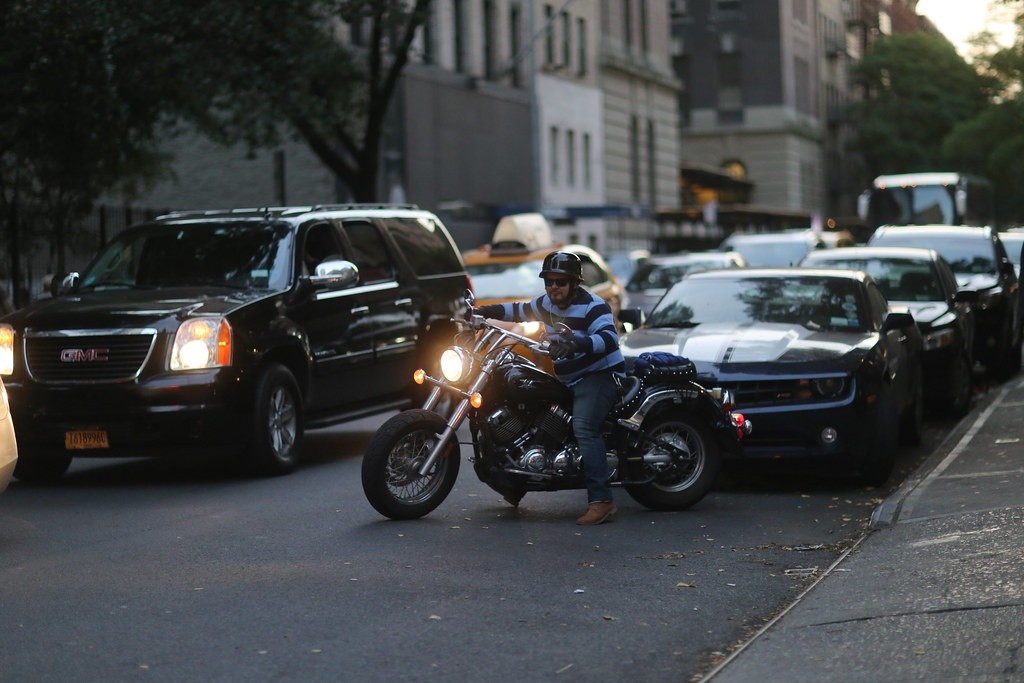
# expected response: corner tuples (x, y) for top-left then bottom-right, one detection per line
(0, 204), (477, 483)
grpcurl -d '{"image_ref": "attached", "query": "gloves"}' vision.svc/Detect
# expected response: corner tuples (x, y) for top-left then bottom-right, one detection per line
(463, 305), (489, 320)
(547, 341), (580, 361)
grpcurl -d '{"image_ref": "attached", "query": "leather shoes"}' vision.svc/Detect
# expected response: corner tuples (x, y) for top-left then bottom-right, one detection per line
(575, 502), (619, 525)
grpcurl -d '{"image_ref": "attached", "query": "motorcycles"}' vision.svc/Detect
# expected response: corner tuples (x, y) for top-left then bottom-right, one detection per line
(361, 289), (752, 520)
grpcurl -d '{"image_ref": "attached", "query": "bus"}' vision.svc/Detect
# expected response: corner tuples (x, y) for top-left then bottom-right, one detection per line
(858, 172), (998, 243)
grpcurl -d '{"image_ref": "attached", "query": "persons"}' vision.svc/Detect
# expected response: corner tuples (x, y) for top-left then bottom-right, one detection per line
(465, 250), (626, 525)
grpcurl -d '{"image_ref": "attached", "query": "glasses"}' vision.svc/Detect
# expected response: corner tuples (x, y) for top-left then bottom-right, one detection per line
(542, 278), (571, 286)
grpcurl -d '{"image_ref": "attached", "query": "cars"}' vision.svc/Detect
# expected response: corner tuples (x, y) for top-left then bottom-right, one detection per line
(863, 223), (1020, 364)
(719, 229), (826, 271)
(997, 233), (1024, 279)
(617, 269), (927, 485)
(430, 212), (625, 400)
(795, 248), (979, 426)
(619, 251), (745, 319)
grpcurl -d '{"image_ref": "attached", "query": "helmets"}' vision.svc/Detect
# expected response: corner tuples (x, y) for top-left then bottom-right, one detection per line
(539, 250), (584, 282)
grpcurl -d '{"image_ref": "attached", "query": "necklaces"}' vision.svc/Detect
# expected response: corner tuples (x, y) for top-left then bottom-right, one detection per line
(549, 301), (570, 331)
